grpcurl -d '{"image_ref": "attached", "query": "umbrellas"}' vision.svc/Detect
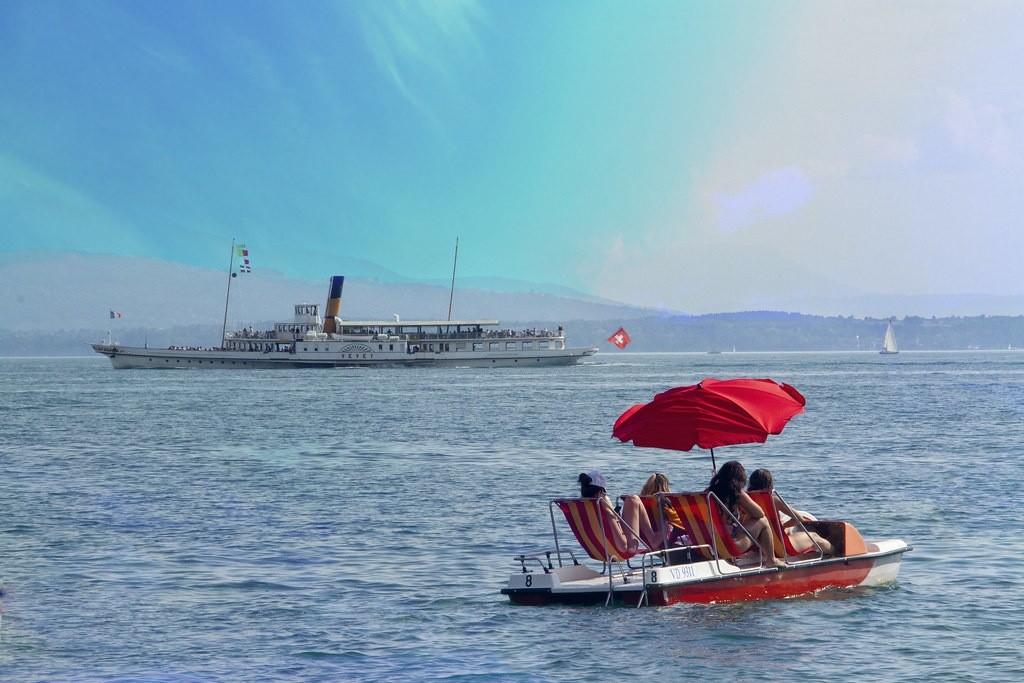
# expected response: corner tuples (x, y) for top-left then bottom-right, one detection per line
(610, 377), (806, 478)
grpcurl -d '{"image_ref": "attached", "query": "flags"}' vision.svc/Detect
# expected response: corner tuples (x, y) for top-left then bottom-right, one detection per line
(110, 311), (121, 319)
(608, 328), (631, 350)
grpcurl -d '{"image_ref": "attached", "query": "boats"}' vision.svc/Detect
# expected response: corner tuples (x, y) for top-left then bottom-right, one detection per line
(87, 233), (601, 371)
(499, 377), (916, 608)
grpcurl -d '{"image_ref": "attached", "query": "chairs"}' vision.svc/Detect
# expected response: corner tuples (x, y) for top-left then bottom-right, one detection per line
(549, 488), (823, 575)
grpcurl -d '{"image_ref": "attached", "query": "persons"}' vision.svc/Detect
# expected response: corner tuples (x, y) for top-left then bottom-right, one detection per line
(578, 460), (838, 568)
(167, 325), (563, 354)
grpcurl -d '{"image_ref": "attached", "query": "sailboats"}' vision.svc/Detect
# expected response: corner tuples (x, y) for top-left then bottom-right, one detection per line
(878, 319), (901, 355)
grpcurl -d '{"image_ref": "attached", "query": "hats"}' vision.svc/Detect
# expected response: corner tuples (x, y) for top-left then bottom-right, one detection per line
(588, 470), (608, 493)
